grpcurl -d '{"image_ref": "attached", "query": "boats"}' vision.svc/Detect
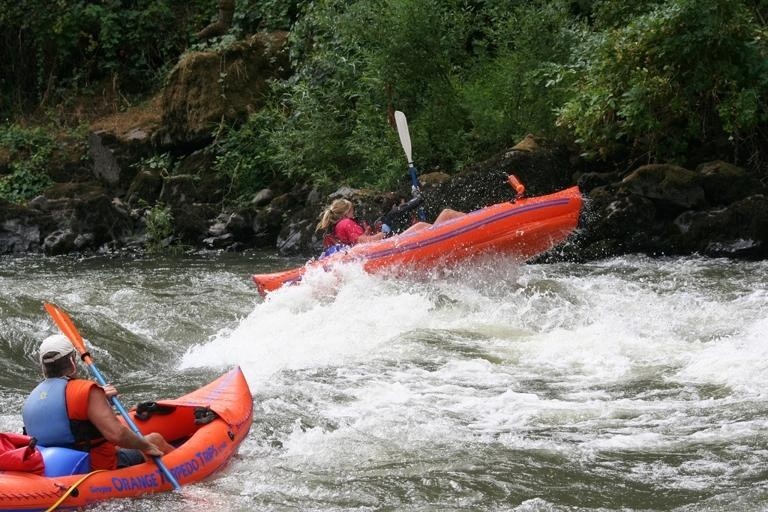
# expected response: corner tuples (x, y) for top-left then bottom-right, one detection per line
(251, 184), (583, 299)
(0, 365), (253, 512)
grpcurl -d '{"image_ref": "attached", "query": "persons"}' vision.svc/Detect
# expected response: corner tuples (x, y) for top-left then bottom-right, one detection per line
(317, 196), (386, 258)
(19, 333), (177, 483)
(374, 189), (465, 239)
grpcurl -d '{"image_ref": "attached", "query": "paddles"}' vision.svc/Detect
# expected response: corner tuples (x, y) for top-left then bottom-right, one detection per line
(395, 110), (427, 223)
(44, 303), (181, 490)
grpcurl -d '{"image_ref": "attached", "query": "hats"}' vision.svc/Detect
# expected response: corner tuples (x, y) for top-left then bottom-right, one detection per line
(38, 333), (77, 363)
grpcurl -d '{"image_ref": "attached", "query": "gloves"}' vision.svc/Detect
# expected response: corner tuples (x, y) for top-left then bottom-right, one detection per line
(381, 224), (391, 236)
(410, 184), (422, 199)
